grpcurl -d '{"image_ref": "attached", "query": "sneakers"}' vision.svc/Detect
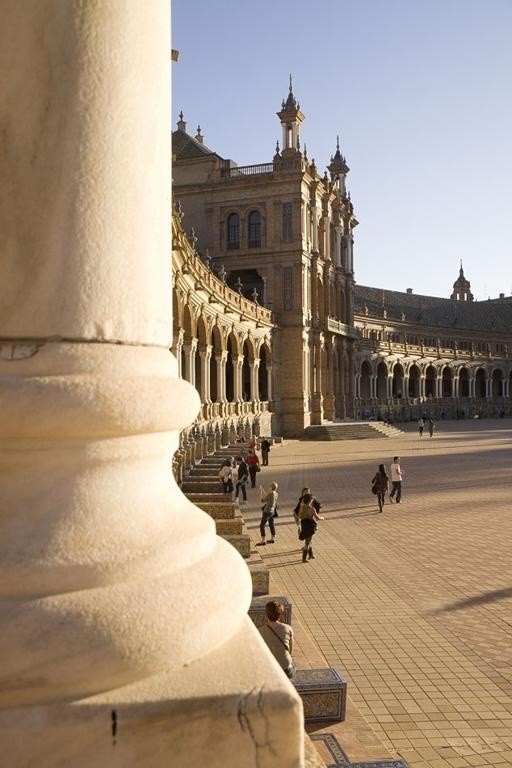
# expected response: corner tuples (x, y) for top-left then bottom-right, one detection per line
(256, 540), (274, 546)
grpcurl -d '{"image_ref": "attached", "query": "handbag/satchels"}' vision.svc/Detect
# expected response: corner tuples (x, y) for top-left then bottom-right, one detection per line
(262, 504), (279, 518)
(372, 486), (376, 495)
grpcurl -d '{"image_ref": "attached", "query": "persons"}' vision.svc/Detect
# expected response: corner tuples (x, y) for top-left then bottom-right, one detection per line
(417, 417), (424, 436)
(293, 486), (322, 550)
(254, 480), (278, 546)
(298, 493), (325, 563)
(371, 463), (390, 514)
(219, 431), (272, 505)
(428, 417), (435, 438)
(388, 456), (406, 504)
(259, 600), (296, 683)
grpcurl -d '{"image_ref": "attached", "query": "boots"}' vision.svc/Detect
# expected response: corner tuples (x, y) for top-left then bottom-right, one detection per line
(303, 547), (315, 563)
